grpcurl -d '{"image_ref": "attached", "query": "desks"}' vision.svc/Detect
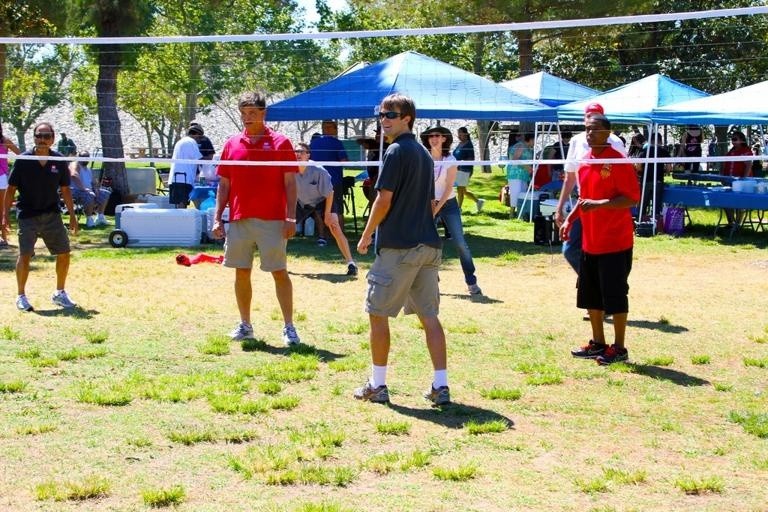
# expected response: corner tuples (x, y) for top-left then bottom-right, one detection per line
(660, 174), (768, 240)
(188, 186), (217, 201)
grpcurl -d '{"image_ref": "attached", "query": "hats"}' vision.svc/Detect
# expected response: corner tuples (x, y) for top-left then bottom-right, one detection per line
(420, 126), (452, 149)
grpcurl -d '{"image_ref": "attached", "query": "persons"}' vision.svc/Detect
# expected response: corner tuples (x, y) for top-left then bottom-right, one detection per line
(618, 133), (674, 219)
(0, 124), (215, 260)
(410, 123), (486, 297)
(751, 128), (767, 178)
(1, 123), (82, 310)
(720, 131), (753, 228)
(505, 130), (572, 221)
(708, 137), (718, 171)
(673, 123), (704, 185)
(294, 143), (358, 271)
(335, 90), (459, 409)
(355, 126), (391, 185)
(212, 87), (305, 353)
(558, 118), (641, 368)
(308, 119), (349, 247)
(410, 119), (486, 297)
(453, 126), (484, 215)
(549, 102), (633, 322)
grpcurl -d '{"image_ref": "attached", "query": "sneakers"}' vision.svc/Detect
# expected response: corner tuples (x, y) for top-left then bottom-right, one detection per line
(86, 213), (111, 227)
(318, 238), (328, 246)
(570, 340), (609, 359)
(52, 290), (76, 306)
(467, 283), (480, 293)
(423, 382), (450, 404)
(346, 264), (358, 275)
(17, 295), (33, 311)
(353, 386), (389, 402)
(477, 200), (483, 211)
(597, 344), (628, 365)
(230, 322), (254, 341)
(282, 325), (300, 346)
(583, 312), (609, 319)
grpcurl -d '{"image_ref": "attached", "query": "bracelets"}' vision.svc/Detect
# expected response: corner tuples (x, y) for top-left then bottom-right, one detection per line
(285, 217), (296, 222)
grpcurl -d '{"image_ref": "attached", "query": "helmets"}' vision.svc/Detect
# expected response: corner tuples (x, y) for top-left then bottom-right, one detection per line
(188, 125), (204, 135)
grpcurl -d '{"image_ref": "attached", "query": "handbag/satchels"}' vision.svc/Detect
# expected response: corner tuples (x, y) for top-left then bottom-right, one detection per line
(636, 222), (657, 236)
(664, 200), (684, 236)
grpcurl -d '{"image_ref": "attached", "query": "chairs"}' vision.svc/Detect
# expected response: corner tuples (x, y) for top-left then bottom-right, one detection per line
(58, 187), (83, 221)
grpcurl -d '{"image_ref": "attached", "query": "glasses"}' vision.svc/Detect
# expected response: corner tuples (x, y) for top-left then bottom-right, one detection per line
(379, 112), (401, 119)
(429, 135), (440, 137)
(34, 132), (52, 140)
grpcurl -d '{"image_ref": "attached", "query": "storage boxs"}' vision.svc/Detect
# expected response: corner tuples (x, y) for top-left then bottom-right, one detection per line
(517, 190), (553, 218)
(663, 207), (684, 234)
(115, 202), (158, 228)
(206, 208), (229, 239)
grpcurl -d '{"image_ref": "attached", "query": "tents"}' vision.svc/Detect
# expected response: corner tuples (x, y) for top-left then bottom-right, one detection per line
(649, 79), (768, 236)
(266, 53), (559, 177)
(518, 73), (723, 237)
(496, 72), (601, 164)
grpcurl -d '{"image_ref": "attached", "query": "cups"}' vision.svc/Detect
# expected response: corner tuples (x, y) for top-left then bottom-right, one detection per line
(200, 177), (206, 186)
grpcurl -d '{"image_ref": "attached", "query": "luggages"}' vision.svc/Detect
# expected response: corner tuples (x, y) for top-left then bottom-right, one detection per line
(170, 172), (192, 205)
